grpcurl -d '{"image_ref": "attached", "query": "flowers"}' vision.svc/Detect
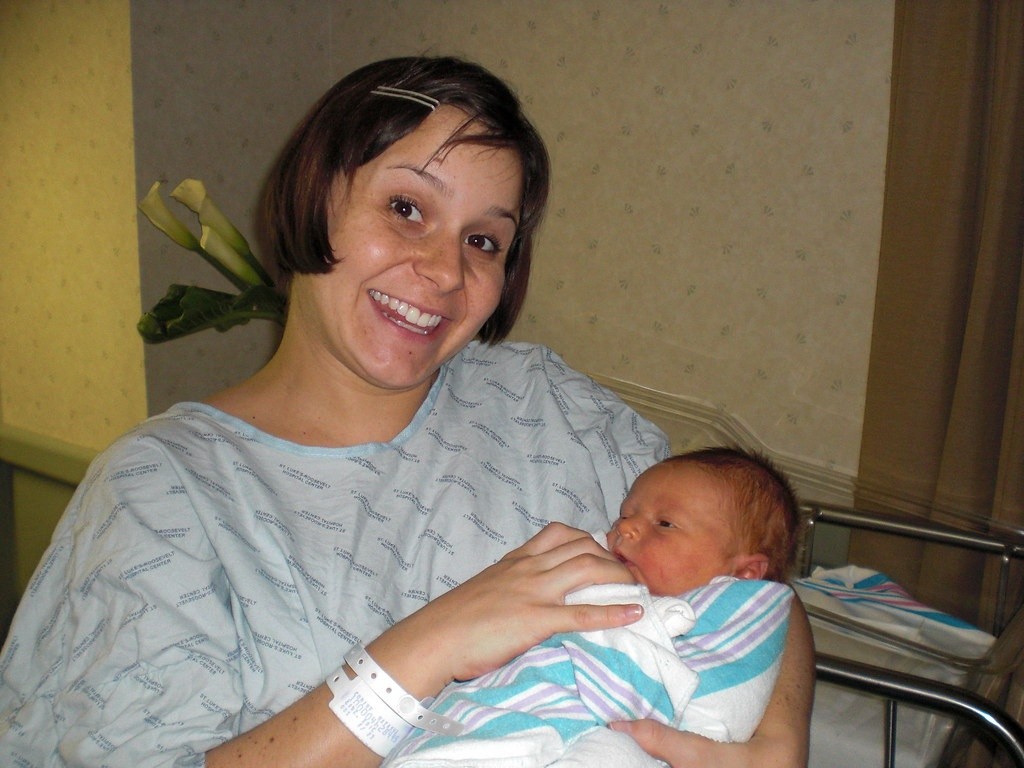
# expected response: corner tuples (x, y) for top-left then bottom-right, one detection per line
(137, 178), (288, 344)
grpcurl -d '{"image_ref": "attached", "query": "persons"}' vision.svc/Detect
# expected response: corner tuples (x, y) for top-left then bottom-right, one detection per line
(0, 50), (816, 768)
(383, 446), (800, 768)
